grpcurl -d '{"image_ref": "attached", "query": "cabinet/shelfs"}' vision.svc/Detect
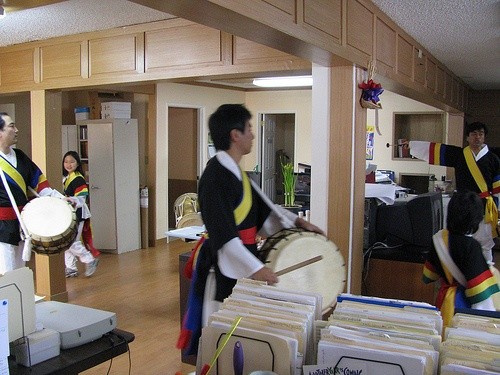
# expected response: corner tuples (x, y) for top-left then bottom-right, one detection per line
(61, 117), (141, 253)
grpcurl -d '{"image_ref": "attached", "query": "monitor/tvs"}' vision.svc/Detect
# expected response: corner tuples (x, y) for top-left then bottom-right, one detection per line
(400, 173), (435, 191)
(377, 191), (443, 257)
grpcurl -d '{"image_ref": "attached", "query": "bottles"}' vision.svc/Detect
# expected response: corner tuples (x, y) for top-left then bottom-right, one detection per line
(399, 139), (408, 158)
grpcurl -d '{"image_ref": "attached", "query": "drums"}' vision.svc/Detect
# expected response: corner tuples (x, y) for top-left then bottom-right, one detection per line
(20, 196), (79, 255)
(173, 192), (204, 230)
(258, 228), (346, 315)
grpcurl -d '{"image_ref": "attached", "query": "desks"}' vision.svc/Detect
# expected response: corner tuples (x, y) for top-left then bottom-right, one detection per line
(368, 257), (437, 305)
(0, 295), (136, 375)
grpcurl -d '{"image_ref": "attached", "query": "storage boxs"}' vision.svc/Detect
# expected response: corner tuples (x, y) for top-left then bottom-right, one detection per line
(74, 89), (132, 120)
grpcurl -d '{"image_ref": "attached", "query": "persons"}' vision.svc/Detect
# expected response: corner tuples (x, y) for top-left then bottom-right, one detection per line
(0, 112), (79, 274)
(175, 104), (327, 356)
(62, 150), (100, 278)
(421, 190), (500, 311)
(403, 123), (500, 265)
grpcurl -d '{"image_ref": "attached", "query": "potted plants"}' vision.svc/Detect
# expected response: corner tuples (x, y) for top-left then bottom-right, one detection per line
(279, 161), (303, 214)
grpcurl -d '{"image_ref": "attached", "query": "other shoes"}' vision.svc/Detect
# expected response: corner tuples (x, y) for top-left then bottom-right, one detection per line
(65, 271), (80, 278)
(84, 256), (100, 277)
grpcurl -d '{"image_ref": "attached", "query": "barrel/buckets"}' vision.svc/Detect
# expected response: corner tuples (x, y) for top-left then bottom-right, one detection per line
(74, 107), (89, 125)
